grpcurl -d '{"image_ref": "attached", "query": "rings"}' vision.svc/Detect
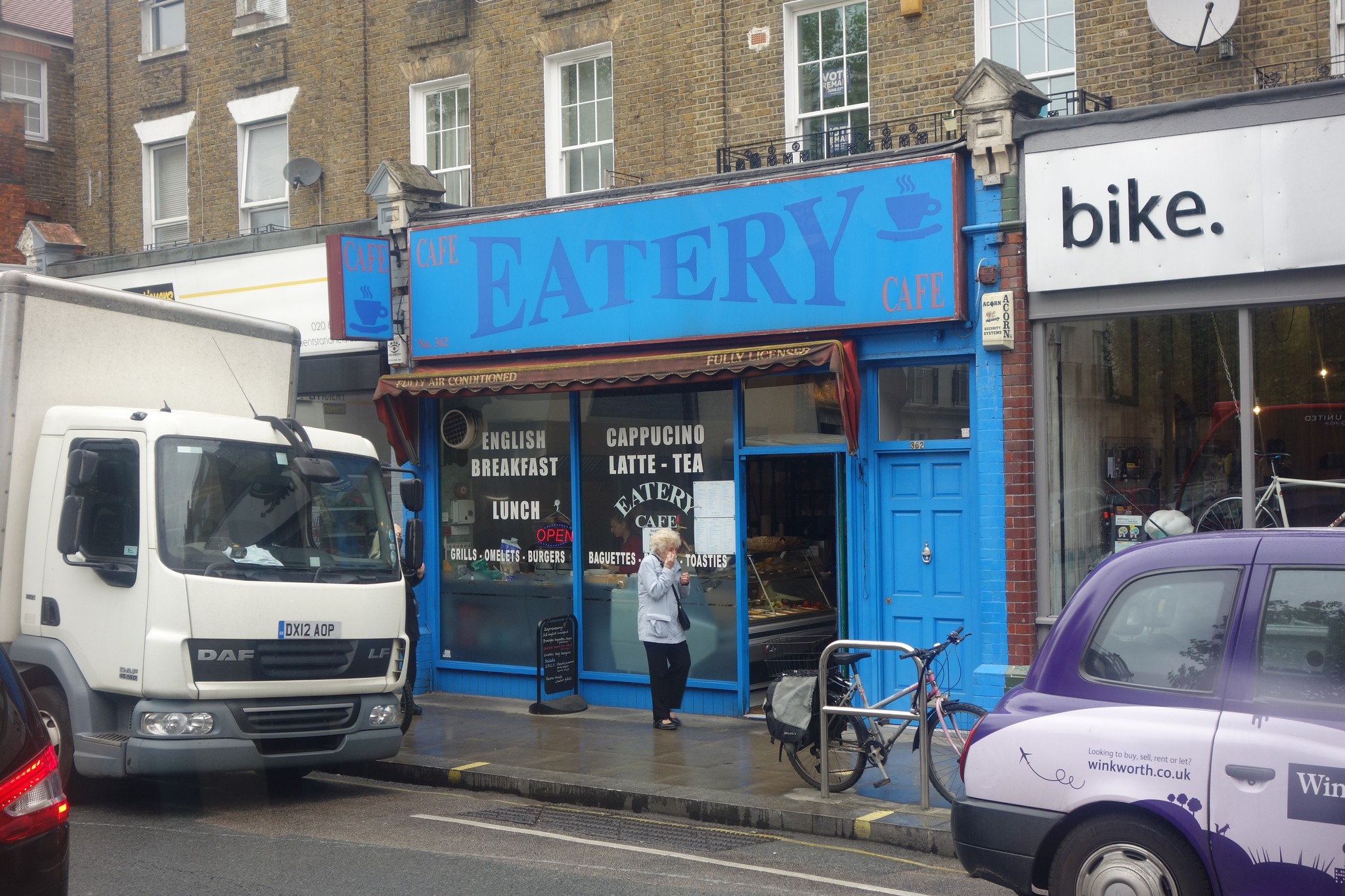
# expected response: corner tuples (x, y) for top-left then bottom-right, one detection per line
(688, 575), (690, 578)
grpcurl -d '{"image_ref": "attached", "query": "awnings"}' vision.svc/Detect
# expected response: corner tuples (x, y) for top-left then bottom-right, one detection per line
(372, 338), (862, 471)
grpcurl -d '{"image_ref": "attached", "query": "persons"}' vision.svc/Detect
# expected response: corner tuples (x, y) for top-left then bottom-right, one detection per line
(637, 528), (691, 730)
(519, 515), (572, 561)
(594, 511), (644, 575)
(366, 524), (427, 715)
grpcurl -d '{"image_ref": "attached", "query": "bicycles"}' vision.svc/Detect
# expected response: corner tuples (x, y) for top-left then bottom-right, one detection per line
(1193, 452), (1345, 532)
(765, 627), (990, 805)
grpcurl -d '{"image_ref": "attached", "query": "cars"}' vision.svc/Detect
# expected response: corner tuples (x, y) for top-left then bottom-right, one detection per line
(1052, 479), (1237, 533)
(950, 526), (1345, 896)
(0, 645), (69, 896)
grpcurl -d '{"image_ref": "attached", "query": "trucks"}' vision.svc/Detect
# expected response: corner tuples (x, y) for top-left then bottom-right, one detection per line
(0, 269), (410, 800)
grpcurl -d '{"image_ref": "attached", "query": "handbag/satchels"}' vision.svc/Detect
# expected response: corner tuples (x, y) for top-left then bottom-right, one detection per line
(678, 603), (691, 631)
(412, 599), (419, 615)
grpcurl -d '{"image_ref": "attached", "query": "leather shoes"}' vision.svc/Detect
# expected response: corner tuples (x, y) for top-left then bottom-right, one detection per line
(400, 697), (422, 715)
(653, 719), (678, 730)
(668, 710), (680, 726)
